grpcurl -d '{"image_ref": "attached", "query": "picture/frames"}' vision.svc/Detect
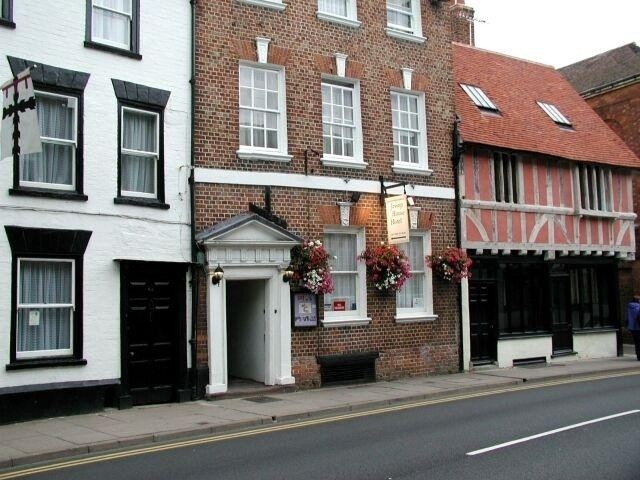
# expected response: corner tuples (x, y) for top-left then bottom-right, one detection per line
(291, 289), (320, 329)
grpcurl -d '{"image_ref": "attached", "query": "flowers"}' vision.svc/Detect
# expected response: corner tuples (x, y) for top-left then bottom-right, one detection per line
(282, 239), (338, 295)
(424, 245), (474, 284)
(355, 245), (417, 297)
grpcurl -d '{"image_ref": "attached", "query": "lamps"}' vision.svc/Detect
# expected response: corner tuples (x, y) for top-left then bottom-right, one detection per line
(282, 264), (296, 286)
(213, 262), (224, 288)
